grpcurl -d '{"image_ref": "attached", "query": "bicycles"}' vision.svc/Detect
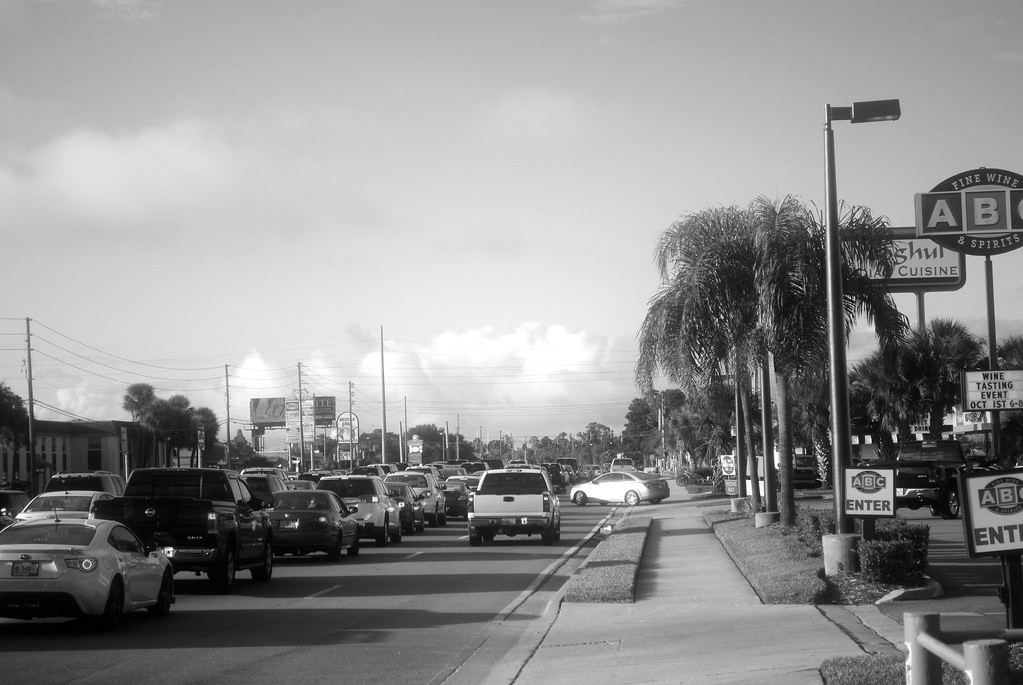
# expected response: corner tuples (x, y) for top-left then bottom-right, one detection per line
(676, 468), (704, 487)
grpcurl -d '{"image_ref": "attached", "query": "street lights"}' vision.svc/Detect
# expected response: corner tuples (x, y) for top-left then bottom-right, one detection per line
(440, 433), (444, 460)
(823, 98), (902, 536)
(710, 375), (746, 498)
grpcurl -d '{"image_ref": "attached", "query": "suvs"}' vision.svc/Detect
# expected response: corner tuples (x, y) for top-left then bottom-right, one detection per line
(42, 470), (128, 498)
(383, 471), (447, 528)
(308, 473), (403, 548)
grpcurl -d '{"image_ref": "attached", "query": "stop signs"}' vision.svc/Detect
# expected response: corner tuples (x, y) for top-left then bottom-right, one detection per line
(198, 431), (205, 450)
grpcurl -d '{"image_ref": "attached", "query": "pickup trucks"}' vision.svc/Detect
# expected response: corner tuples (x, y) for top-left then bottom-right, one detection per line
(88, 467), (275, 593)
(858, 439), (999, 520)
(467, 468), (561, 548)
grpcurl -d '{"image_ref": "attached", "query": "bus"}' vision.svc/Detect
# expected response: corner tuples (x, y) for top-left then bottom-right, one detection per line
(617, 452), (644, 471)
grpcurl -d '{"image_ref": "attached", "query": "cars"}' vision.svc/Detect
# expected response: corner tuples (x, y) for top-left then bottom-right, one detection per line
(777, 453), (822, 491)
(610, 458), (636, 472)
(570, 471), (670, 506)
(240, 467), (295, 481)
(384, 482), (426, 535)
(14, 491), (115, 521)
(299, 458), (612, 489)
(284, 480), (318, 490)
(240, 474), (297, 503)
(1, 519), (174, 635)
(261, 490), (361, 562)
(439, 482), (473, 521)
